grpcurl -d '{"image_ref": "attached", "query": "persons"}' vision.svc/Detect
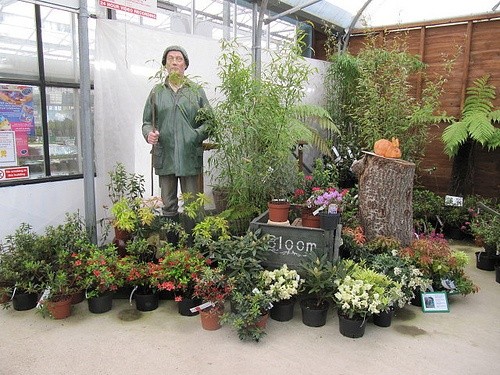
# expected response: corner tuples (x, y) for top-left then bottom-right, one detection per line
(142, 45), (214, 249)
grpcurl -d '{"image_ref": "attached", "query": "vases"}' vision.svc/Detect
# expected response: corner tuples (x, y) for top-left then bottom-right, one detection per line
(200, 309), (222, 330)
(133, 292), (156, 310)
(272, 301), (295, 322)
(87, 291), (112, 314)
(338, 312), (367, 340)
(474, 235), (484, 247)
(245, 310), (269, 331)
(177, 297), (199, 317)
(373, 310), (395, 328)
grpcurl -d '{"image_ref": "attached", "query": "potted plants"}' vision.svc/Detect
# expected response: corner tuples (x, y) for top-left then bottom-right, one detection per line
(295, 251), (338, 327)
(97, 16), (320, 251)
(318, 8), (466, 250)
(0, 213), (85, 321)
(475, 204), (500, 283)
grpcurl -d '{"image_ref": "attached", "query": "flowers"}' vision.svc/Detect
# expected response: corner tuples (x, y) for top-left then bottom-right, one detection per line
(71, 245), (306, 342)
(333, 251), (433, 328)
(412, 230), (454, 260)
(461, 208), (491, 239)
